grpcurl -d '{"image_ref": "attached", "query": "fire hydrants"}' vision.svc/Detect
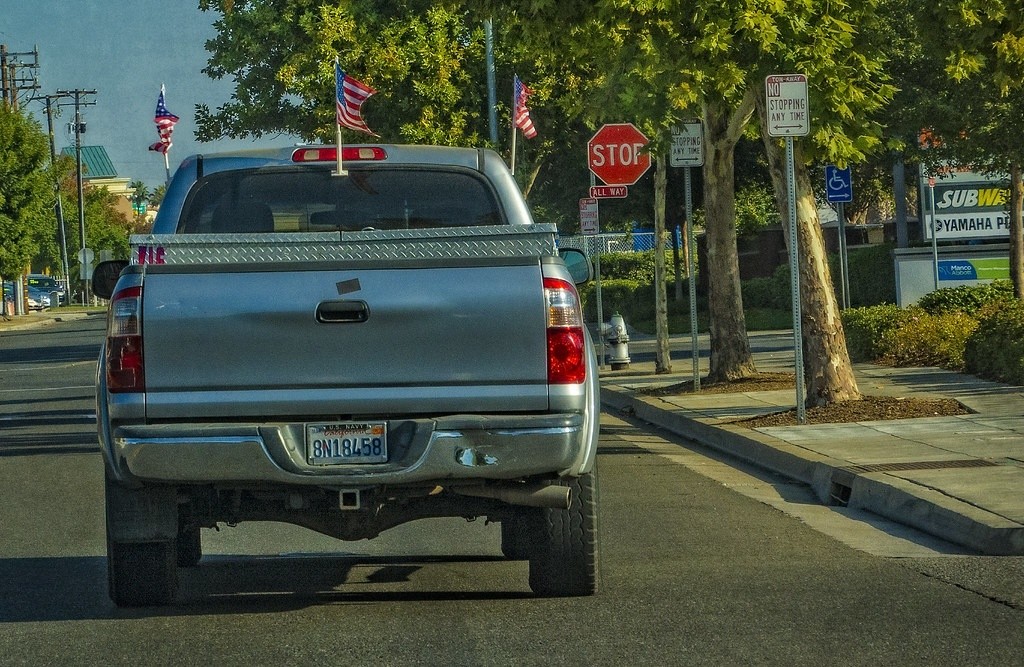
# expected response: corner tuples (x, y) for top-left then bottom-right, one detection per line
(599, 310), (632, 370)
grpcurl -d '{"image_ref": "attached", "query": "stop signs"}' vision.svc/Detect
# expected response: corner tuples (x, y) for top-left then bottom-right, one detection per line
(587, 123), (652, 186)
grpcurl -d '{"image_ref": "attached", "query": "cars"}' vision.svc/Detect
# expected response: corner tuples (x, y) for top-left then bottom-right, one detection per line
(26, 274), (67, 308)
(3, 282), (52, 310)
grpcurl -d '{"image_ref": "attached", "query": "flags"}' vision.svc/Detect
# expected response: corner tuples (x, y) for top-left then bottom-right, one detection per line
(335, 61), (381, 138)
(148, 90), (180, 156)
(514, 74), (538, 140)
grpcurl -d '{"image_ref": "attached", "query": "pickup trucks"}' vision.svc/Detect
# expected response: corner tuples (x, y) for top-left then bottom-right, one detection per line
(96, 141), (601, 606)
